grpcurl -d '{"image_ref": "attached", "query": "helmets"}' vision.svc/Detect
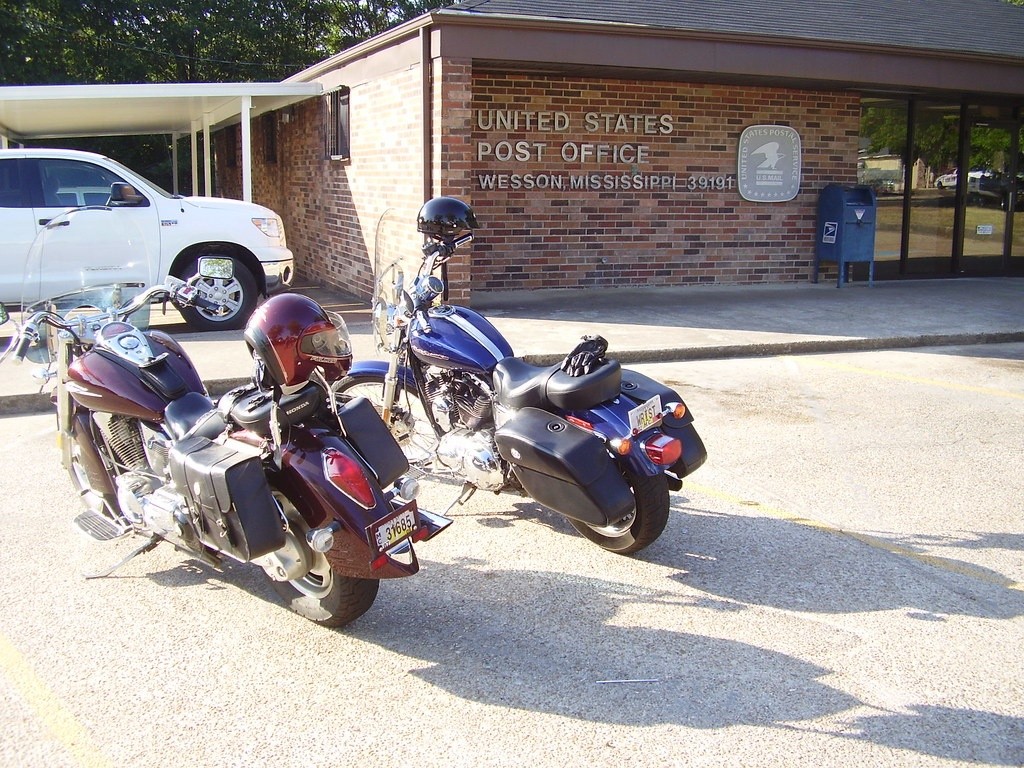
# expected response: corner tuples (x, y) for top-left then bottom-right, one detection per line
(244, 293), (352, 395)
(418, 197), (481, 235)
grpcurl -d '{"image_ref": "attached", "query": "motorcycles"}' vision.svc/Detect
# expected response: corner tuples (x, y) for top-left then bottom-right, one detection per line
(0, 207), (456, 629)
(327, 196), (710, 557)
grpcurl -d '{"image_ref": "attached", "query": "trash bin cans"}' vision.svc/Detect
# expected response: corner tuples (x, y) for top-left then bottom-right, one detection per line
(813, 184), (877, 290)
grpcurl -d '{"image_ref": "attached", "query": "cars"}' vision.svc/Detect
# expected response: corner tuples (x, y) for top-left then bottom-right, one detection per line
(934, 163), (1024, 212)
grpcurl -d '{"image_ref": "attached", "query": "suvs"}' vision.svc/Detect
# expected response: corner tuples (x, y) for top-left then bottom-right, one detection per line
(0, 147), (297, 333)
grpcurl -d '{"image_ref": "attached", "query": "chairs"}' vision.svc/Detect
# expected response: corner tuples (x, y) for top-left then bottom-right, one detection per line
(46, 177), (63, 206)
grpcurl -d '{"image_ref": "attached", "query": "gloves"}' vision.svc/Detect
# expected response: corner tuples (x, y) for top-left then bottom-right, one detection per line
(560, 333), (607, 377)
